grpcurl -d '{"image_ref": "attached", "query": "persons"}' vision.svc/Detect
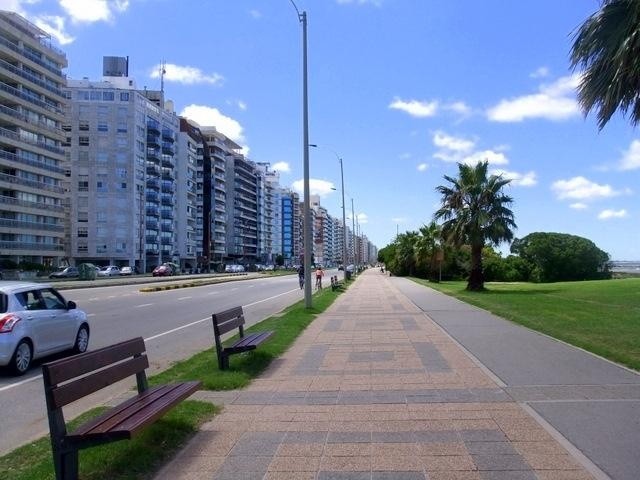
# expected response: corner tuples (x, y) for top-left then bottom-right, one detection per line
(314, 268), (323, 288)
(296, 264), (305, 289)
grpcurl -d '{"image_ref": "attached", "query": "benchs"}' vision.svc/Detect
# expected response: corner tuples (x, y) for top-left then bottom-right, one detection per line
(334, 275), (346, 292)
(210, 306), (278, 373)
(331, 277), (342, 293)
(38, 331), (206, 475)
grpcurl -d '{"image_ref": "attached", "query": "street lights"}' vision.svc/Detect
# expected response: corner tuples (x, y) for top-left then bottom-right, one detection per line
(330, 185), (363, 262)
(309, 143), (347, 271)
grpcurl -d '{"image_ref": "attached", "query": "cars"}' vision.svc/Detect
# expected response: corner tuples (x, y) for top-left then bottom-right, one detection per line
(152, 265), (173, 277)
(224, 263), (325, 273)
(49, 261), (137, 277)
(336, 262), (365, 274)
(0, 279), (90, 377)
